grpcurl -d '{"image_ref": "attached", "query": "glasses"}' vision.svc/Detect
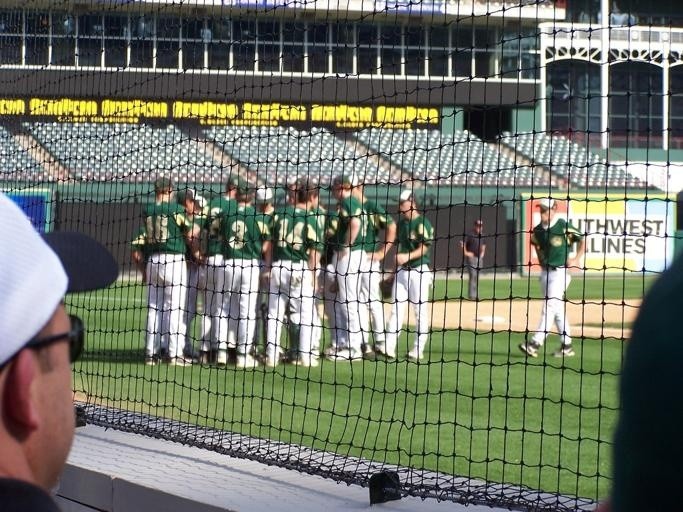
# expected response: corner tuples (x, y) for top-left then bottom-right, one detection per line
(0, 314), (85, 374)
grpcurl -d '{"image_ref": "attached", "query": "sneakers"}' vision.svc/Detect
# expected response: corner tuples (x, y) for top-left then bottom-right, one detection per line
(143, 349), (281, 368)
(518, 340), (541, 358)
(287, 341), (424, 367)
(550, 345), (575, 357)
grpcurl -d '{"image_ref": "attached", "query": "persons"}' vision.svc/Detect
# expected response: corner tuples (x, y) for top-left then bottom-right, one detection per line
(518, 196), (586, 360)
(1, 189), (120, 512)
(128, 170), (436, 372)
(459, 218), (487, 300)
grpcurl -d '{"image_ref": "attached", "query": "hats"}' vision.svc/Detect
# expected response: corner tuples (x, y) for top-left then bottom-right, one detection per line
(153, 175), (273, 208)
(0, 191), (120, 374)
(536, 198), (556, 210)
(284, 171), (416, 203)
